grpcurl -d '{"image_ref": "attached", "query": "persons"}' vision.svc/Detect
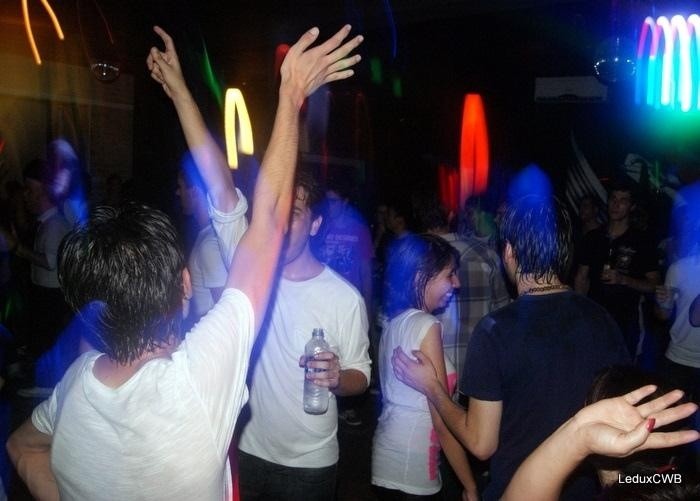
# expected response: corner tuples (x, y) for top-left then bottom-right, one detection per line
(0, 22), (700, 501)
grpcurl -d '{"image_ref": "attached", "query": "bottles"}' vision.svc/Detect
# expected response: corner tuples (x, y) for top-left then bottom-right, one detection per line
(303, 327), (332, 415)
(600, 248), (611, 284)
